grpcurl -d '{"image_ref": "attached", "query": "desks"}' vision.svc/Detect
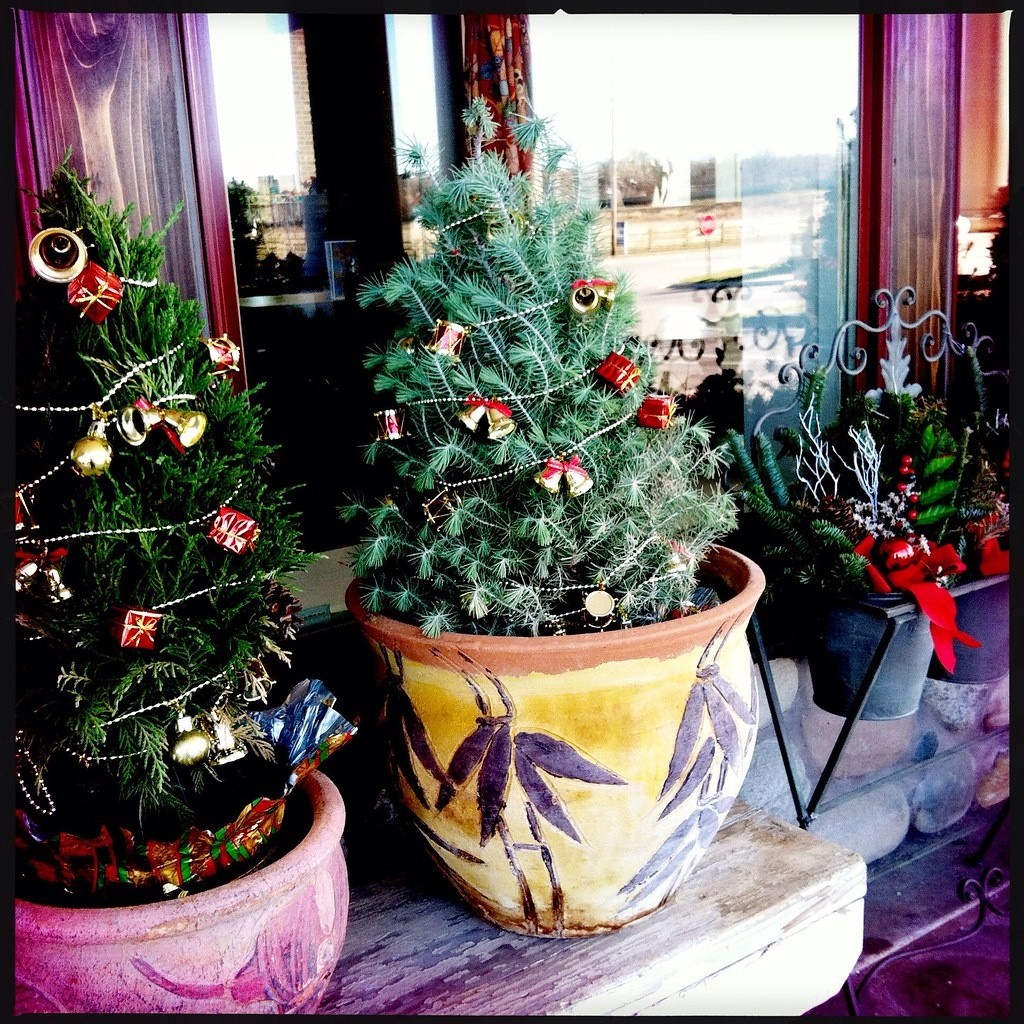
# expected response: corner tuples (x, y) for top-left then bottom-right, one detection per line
(308, 799), (866, 1024)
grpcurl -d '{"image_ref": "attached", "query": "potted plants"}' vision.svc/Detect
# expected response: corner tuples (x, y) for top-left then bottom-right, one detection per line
(0, 100), (1008, 1016)
